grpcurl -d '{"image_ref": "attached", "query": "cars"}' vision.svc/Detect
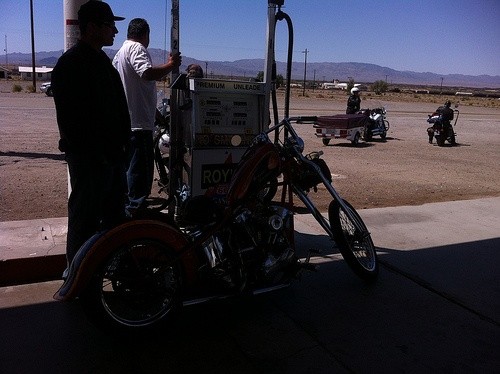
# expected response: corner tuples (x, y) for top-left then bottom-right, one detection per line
(40, 82), (53, 97)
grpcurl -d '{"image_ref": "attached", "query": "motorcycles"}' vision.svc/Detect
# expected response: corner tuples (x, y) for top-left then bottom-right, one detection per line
(427, 104), (459, 147)
(155, 97), (191, 195)
(52, 117), (378, 344)
(313, 107), (389, 146)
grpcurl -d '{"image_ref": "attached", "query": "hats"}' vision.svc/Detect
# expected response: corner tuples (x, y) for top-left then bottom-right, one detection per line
(78, 1), (125, 26)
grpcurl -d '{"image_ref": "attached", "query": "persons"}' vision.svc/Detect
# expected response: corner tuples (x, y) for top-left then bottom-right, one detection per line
(427, 101), (453, 144)
(346, 86), (361, 115)
(112, 17), (182, 221)
(50, 0), (134, 303)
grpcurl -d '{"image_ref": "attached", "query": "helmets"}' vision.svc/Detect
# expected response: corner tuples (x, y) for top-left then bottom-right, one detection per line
(351, 87), (360, 95)
